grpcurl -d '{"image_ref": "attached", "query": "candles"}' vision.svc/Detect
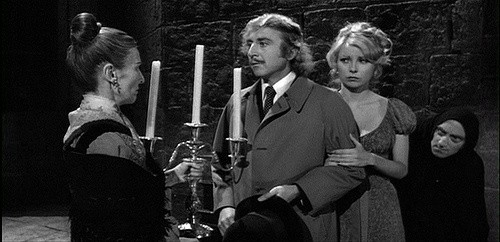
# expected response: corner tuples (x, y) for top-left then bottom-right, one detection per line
(233, 67), (242, 140)
(191, 44), (204, 124)
(144, 58), (161, 139)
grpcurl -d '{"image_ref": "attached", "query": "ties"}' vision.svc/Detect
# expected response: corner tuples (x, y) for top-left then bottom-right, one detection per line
(263, 86), (276, 119)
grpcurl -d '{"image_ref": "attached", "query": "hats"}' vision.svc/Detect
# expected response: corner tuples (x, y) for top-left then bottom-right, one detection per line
(224, 194), (312, 242)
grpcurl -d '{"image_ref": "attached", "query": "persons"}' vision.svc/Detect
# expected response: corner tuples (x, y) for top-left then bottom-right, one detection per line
(211, 13), (366, 242)
(391, 106), (489, 242)
(326, 22), (417, 242)
(61, 13), (193, 242)
